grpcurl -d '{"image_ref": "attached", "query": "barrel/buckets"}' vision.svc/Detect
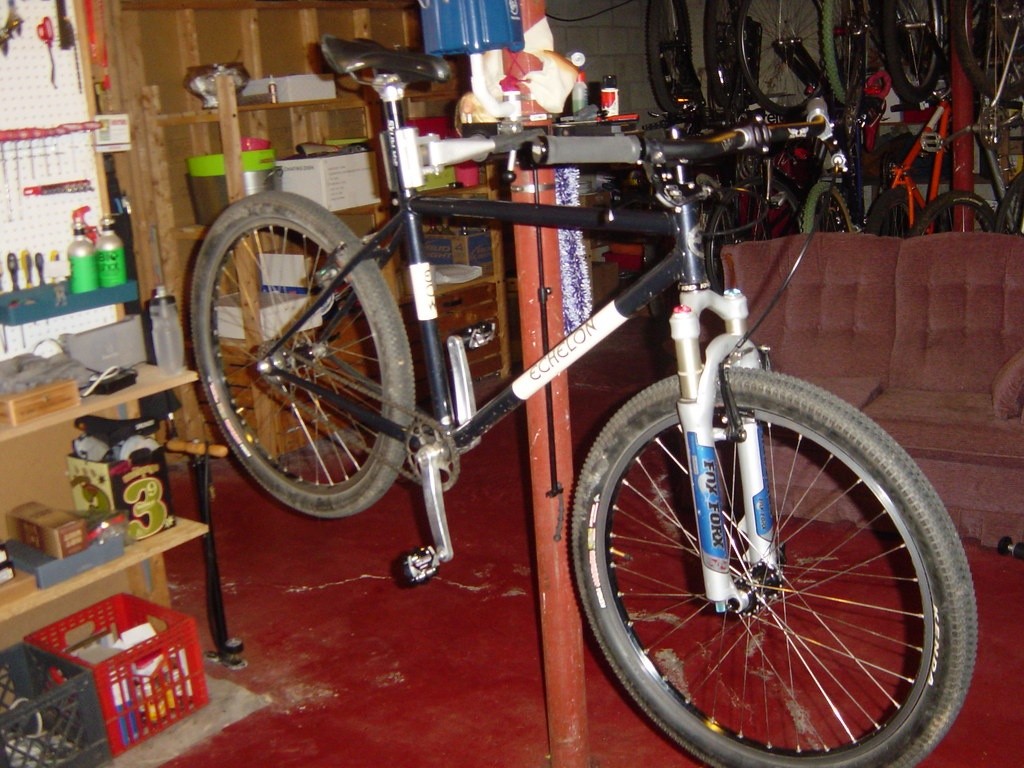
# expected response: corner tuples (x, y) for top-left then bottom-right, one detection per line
(187, 149), (276, 177)
(184, 168), (283, 225)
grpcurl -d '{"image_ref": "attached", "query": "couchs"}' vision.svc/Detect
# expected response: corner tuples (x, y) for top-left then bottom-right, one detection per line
(721, 232), (1024, 549)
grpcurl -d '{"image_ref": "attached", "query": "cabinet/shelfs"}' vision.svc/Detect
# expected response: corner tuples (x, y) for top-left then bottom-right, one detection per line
(141, 73), (511, 460)
(0, 280), (212, 657)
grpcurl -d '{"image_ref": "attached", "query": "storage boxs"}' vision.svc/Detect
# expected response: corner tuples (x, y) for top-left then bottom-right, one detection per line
(0, 531), (126, 589)
(66, 450), (177, 548)
(0, 381), (81, 428)
(240, 72), (336, 103)
(273, 151), (380, 213)
(5, 501), (86, 560)
(0, 567), (37, 607)
(213, 248), (341, 340)
(24, 592), (208, 758)
(422, 225), (494, 277)
(0, 641), (114, 768)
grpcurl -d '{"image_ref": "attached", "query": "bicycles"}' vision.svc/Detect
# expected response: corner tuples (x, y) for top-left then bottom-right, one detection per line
(188, 35), (981, 768)
(600, 2), (1024, 235)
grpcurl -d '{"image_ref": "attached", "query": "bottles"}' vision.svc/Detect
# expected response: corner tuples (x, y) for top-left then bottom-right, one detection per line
(601, 75), (619, 118)
(68, 216), (98, 292)
(149, 286), (184, 376)
(97, 218), (126, 287)
(572, 72), (587, 116)
